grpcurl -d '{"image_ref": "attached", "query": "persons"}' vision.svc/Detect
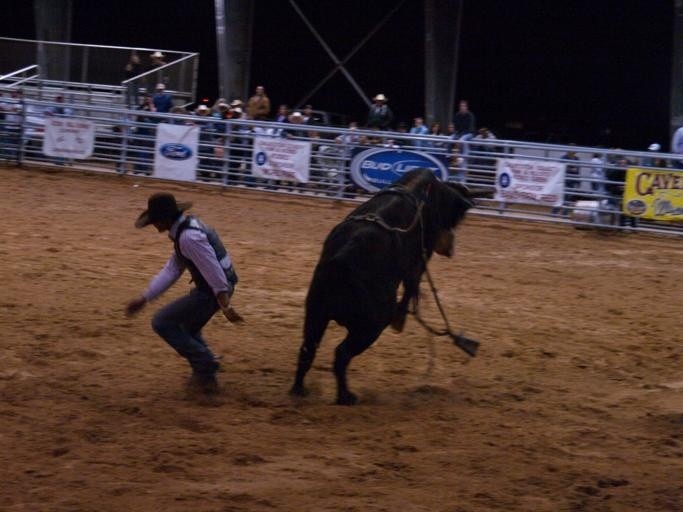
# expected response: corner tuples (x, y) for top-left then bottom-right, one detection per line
(120, 191), (244, 389)
(126, 50), (171, 104)
(130, 83), (495, 198)
(0, 86), (71, 156)
(559, 124), (683, 234)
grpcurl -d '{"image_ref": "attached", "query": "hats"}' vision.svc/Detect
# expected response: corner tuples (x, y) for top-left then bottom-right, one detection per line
(134, 191), (193, 229)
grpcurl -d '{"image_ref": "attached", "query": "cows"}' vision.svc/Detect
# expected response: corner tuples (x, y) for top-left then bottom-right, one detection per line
(287, 167), (498, 407)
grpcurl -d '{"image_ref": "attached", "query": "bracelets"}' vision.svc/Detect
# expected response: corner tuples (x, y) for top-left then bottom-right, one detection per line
(220, 304), (232, 313)
